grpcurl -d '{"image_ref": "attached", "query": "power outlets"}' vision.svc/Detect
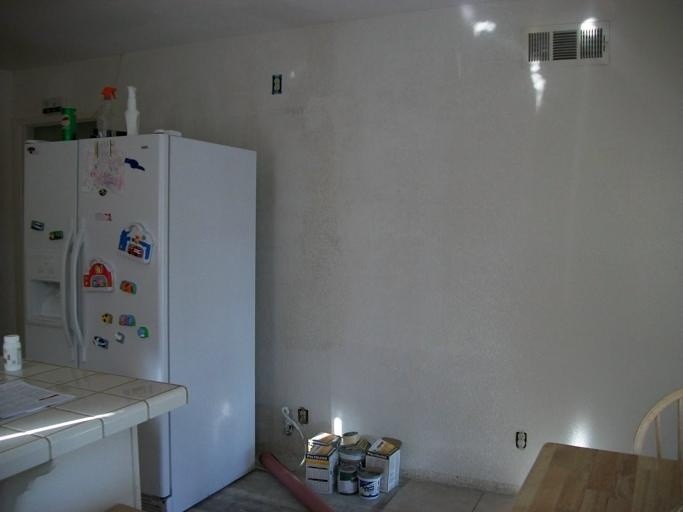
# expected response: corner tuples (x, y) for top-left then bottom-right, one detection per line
(284, 423), (293, 435)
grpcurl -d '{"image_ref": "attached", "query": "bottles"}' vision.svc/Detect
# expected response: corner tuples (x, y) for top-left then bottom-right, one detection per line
(60, 104), (76, 140)
(3, 334), (22, 372)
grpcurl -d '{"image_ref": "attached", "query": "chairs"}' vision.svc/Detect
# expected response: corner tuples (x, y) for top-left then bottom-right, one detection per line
(633, 388), (683, 460)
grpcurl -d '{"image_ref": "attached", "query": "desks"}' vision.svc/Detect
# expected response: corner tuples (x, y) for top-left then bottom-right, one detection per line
(0, 358), (188, 512)
(509, 442), (683, 512)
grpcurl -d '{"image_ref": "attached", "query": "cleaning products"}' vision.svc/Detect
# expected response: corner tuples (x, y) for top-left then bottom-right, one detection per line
(95, 86), (119, 137)
(124, 86), (142, 134)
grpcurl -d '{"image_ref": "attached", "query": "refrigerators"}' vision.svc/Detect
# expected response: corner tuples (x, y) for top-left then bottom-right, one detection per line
(23, 137), (258, 506)
